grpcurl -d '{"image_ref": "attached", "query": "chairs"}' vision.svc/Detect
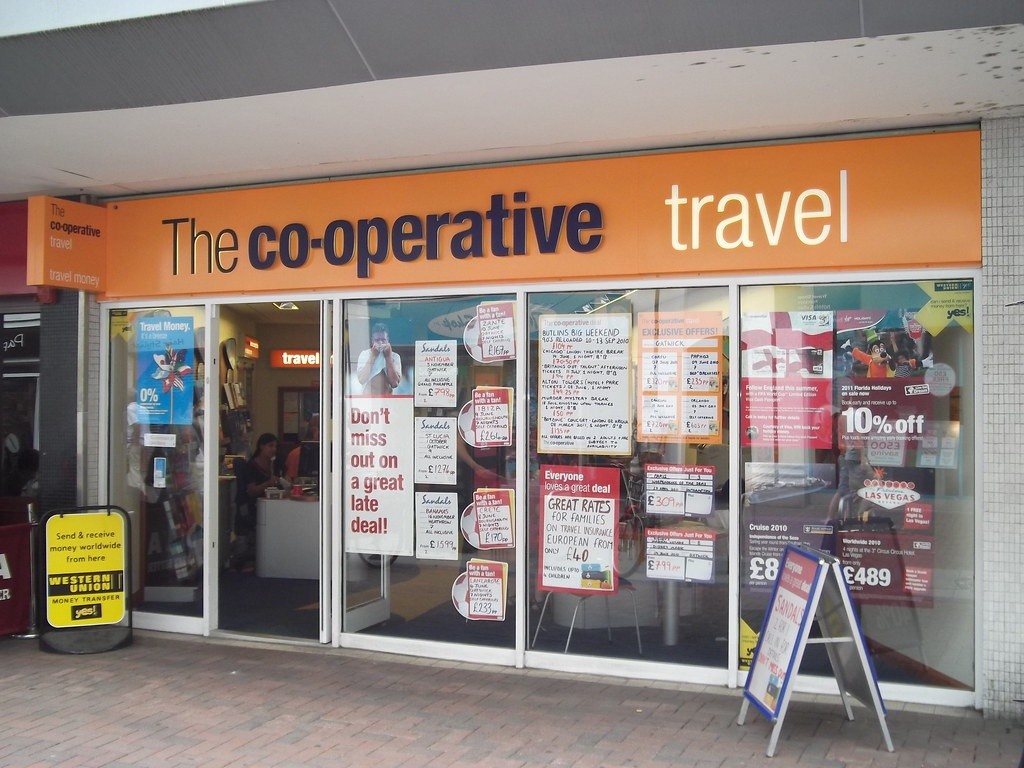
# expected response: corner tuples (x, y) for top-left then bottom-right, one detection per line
(531, 512), (644, 657)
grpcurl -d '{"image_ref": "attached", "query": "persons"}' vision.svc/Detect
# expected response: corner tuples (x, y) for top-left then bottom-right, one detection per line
(127, 380), (200, 585)
(287, 413), (319, 480)
(356, 322), (402, 395)
(238, 433), (292, 531)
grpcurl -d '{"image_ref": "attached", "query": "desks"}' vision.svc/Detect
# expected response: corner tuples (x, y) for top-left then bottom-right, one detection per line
(256, 497), (320, 580)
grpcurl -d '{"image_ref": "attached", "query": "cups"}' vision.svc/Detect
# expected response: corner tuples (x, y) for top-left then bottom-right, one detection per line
(292, 485), (303, 497)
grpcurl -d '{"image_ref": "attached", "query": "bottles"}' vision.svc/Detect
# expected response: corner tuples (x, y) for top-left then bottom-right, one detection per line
(163, 499), (197, 585)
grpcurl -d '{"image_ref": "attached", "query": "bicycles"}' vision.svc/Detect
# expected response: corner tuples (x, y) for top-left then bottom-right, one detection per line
(609, 462), (648, 578)
